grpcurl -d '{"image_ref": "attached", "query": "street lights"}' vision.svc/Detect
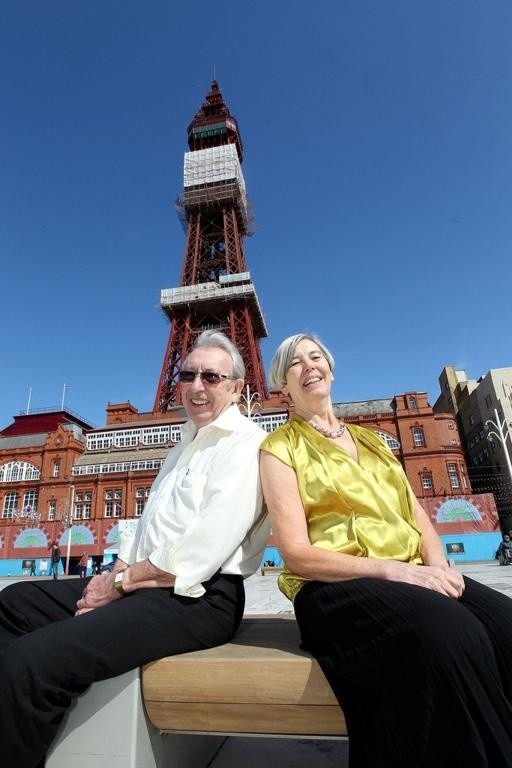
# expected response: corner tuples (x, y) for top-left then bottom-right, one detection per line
(483, 408), (512, 482)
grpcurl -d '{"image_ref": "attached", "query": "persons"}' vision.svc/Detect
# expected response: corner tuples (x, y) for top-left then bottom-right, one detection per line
(0, 327), (269, 768)
(499, 535), (512, 559)
(29, 562), (37, 577)
(91, 560), (103, 575)
(51, 543), (61, 580)
(260, 332), (512, 768)
(76, 551), (88, 578)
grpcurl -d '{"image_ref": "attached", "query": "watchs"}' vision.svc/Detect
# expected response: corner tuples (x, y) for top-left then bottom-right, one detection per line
(114, 568), (129, 596)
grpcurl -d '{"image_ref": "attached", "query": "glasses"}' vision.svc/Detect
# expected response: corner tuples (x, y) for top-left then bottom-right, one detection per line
(177, 367), (236, 388)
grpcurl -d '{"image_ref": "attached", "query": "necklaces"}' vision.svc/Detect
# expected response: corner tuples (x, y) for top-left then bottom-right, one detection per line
(301, 414), (346, 438)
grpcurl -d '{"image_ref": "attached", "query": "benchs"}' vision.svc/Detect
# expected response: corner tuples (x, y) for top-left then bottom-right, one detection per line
(43, 617), (348, 768)
